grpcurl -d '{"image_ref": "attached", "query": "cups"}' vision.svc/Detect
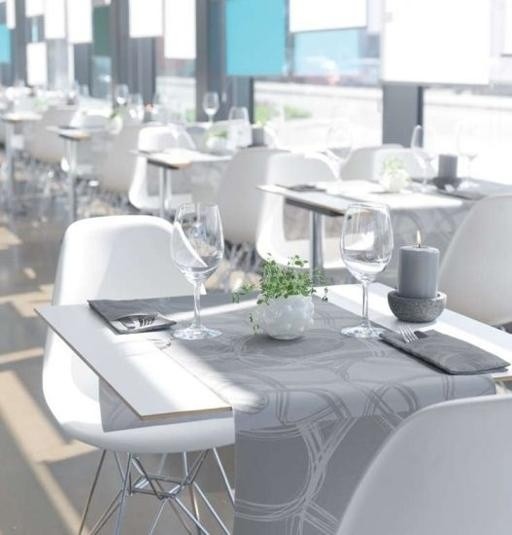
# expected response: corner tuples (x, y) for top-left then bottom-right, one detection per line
(62, 81), (145, 128)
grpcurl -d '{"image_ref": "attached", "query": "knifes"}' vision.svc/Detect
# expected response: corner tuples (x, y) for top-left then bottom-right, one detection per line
(412, 329), (426, 341)
(120, 317), (134, 330)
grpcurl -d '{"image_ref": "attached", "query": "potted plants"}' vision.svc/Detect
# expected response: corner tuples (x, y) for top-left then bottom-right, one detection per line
(233, 250), (331, 344)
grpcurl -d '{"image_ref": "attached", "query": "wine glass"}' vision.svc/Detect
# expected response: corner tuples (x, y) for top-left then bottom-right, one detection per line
(339, 201), (392, 339)
(325, 123), (354, 196)
(170, 201), (223, 342)
(410, 125), (439, 197)
(201, 92), (286, 161)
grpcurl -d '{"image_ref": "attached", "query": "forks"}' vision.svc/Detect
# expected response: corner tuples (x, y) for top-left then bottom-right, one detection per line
(399, 323), (419, 347)
(139, 314), (155, 328)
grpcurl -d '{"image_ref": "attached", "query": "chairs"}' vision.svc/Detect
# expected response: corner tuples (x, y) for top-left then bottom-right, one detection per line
(436, 192), (511, 328)
(336, 391), (510, 530)
(0, 87), (433, 259)
(40, 211), (235, 531)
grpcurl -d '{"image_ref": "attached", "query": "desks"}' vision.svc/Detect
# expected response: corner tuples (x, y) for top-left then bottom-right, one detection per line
(257, 178), (485, 284)
(33, 281), (512, 420)
